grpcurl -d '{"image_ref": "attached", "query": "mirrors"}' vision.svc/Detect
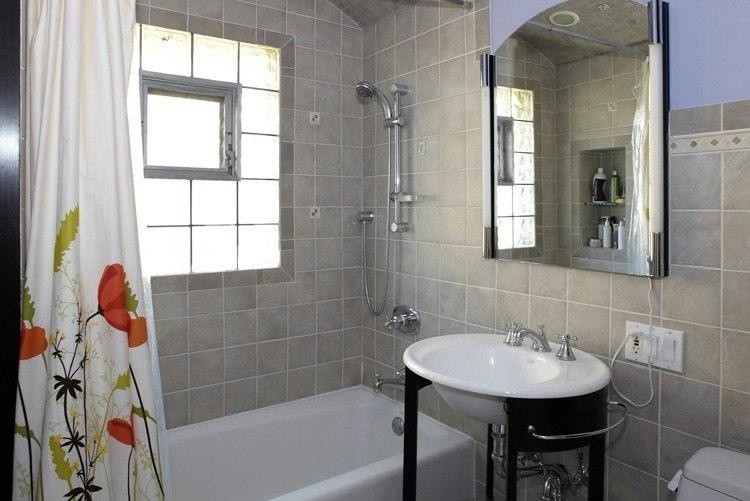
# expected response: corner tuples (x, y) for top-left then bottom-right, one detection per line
(474, 1), (674, 282)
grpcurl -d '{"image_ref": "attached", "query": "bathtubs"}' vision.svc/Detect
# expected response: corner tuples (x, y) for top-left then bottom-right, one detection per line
(165, 382), (476, 500)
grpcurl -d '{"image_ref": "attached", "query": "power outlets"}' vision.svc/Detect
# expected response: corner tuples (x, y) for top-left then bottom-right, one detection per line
(620, 320), (686, 377)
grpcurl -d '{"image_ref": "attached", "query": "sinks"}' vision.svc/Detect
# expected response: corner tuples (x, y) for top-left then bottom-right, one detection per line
(401, 332), (612, 426)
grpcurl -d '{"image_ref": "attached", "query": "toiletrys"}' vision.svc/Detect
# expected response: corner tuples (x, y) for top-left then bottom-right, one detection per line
(588, 216), (625, 250)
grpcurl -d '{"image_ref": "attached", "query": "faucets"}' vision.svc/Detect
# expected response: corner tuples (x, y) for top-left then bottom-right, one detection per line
(512, 323), (552, 352)
